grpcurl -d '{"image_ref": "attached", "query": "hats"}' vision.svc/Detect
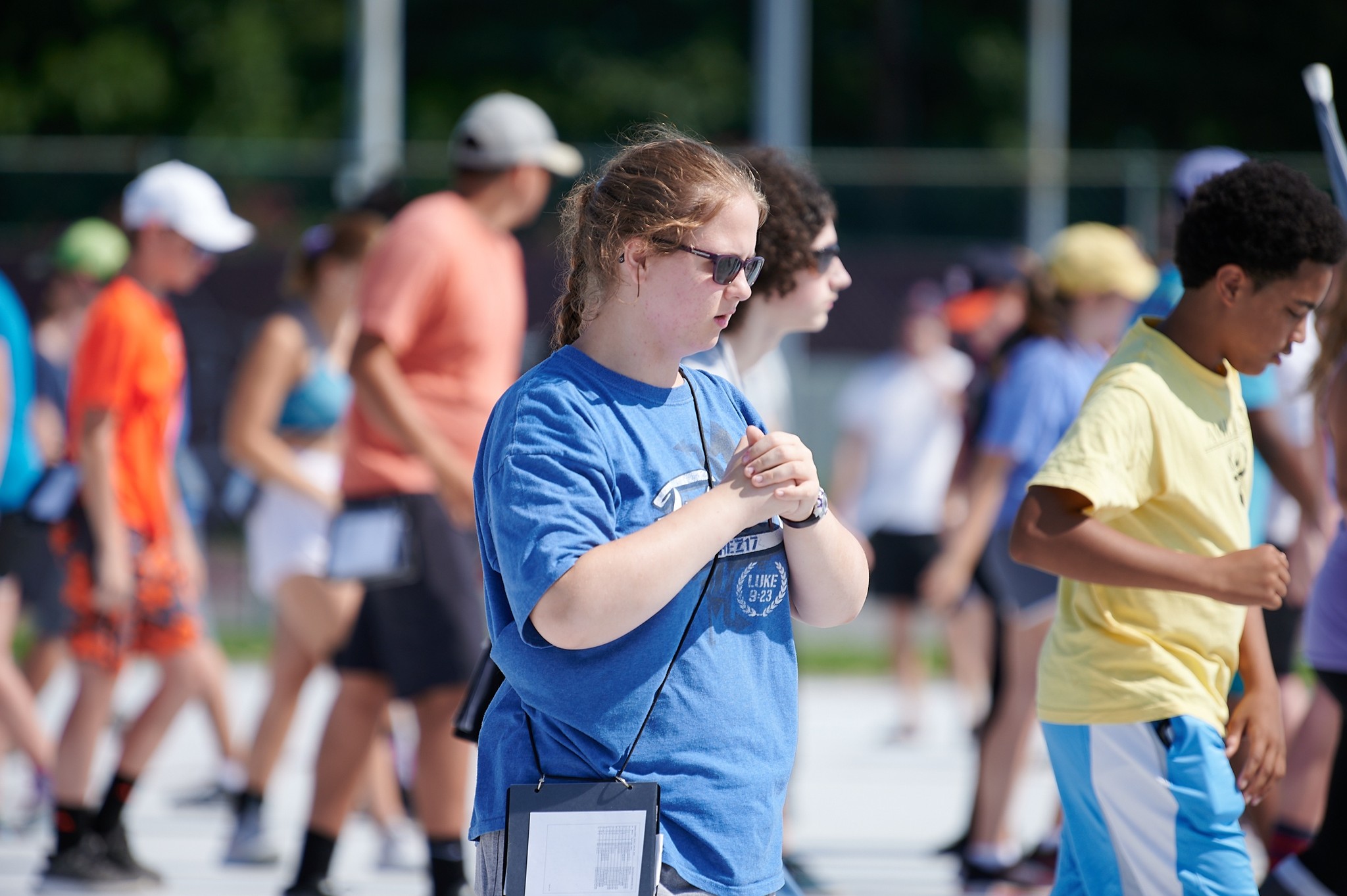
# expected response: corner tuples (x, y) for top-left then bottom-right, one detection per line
(445, 89), (584, 178)
(1028, 220), (1161, 303)
(58, 216), (130, 284)
(1170, 145), (1251, 202)
(121, 158), (257, 252)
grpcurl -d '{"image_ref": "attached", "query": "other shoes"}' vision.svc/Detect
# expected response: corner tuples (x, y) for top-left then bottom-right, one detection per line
(375, 819), (430, 870)
(224, 812), (284, 868)
(958, 837), (1059, 896)
(40, 815), (174, 896)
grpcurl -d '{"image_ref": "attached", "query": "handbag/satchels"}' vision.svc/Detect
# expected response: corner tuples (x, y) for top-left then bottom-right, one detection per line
(499, 774), (664, 896)
(319, 505), (424, 586)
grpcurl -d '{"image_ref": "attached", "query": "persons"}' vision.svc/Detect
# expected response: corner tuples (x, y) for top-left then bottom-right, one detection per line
(678, 148), (854, 405)
(281, 97), (553, 895)
(0, 162), (425, 892)
(1010, 152), (1347, 896)
(474, 137), (871, 896)
(833, 149), (1346, 896)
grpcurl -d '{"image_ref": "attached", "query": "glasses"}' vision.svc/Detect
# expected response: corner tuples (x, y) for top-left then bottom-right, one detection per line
(617, 237), (765, 287)
(791, 245), (840, 273)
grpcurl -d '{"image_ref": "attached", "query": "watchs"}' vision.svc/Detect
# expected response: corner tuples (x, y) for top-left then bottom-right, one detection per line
(779, 486), (829, 529)
